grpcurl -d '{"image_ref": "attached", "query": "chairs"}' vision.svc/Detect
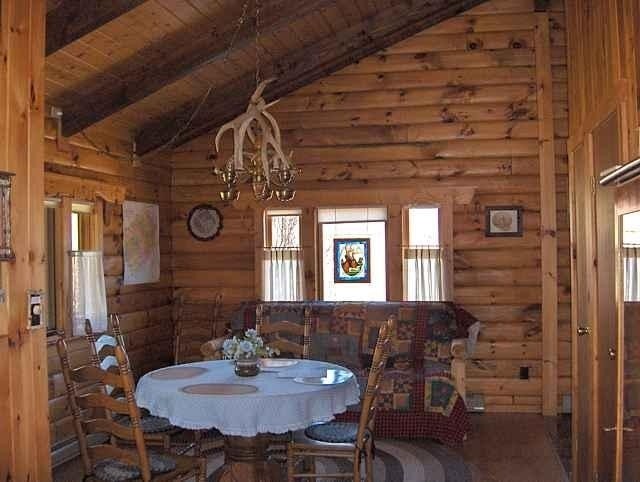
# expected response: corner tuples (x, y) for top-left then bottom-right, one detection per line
(254, 302), (314, 358)
(55, 337), (207, 482)
(172, 294), (222, 364)
(285, 314), (398, 480)
(83, 316), (181, 453)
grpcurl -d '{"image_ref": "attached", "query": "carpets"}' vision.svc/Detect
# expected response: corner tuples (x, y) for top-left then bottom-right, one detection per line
(170, 438), (473, 482)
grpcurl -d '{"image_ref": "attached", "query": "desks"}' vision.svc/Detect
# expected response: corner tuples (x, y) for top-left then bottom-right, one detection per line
(129, 358), (360, 481)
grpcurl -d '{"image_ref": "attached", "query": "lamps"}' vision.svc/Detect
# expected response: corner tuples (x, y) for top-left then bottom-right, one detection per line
(211, 148), (295, 206)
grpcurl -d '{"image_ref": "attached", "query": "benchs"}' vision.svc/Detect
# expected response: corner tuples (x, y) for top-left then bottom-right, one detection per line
(198, 300), (483, 447)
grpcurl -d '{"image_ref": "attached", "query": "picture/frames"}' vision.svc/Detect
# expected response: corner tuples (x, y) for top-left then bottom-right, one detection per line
(183, 203), (224, 241)
(333, 237), (371, 284)
(483, 205), (522, 237)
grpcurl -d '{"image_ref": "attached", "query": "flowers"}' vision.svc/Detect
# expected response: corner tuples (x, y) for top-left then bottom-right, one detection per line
(220, 328), (280, 358)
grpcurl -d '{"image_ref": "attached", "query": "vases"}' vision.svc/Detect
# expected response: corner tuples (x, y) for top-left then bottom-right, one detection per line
(234, 358), (261, 376)
